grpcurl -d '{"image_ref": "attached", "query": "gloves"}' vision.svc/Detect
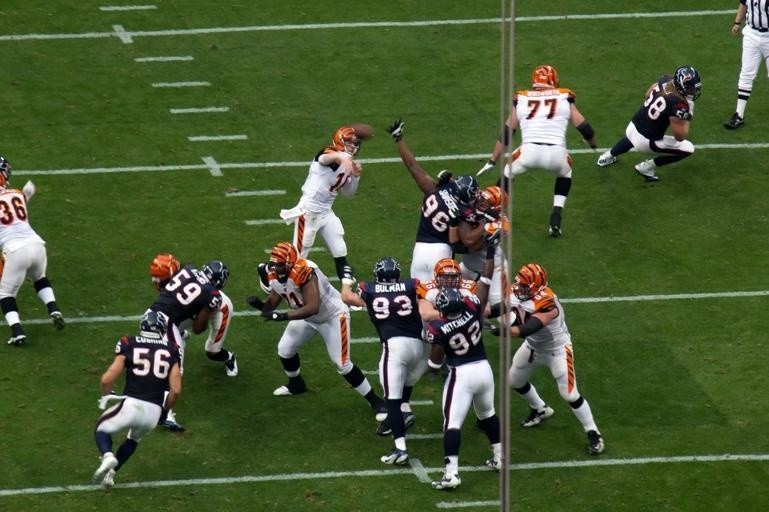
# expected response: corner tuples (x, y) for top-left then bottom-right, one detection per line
(481, 231), (501, 260)
(261, 311), (289, 323)
(474, 159), (497, 178)
(436, 170), (452, 181)
(340, 265), (357, 286)
(246, 295), (265, 310)
(385, 118), (405, 143)
(489, 327), (501, 337)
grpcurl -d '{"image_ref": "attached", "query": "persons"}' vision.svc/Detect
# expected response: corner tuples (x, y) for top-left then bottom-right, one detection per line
(278, 124), (361, 287)
(484, 263), (606, 455)
(245, 242), (387, 421)
(140, 261), (229, 431)
(141, 251), (239, 378)
(340, 257), (428, 466)
(428, 288), (503, 489)
(452, 182), (512, 330)
(414, 257), (480, 321)
(722, 0), (769, 128)
(598, 66), (703, 182)
(0, 158), (66, 344)
(89, 308), (182, 491)
(476, 65), (597, 239)
(384, 117), (487, 289)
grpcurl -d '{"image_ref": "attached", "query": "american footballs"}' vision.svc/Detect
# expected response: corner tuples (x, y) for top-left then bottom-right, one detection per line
(353, 123), (375, 140)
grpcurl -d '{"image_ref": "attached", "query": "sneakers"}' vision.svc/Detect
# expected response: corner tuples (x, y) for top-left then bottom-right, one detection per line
(587, 430), (605, 456)
(431, 472), (461, 490)
(723, 112), (745, 130)
(372, 399), (389, 423)
(91, 451), (119, 485)
(380, 448), (409, 465)
(224, 351), (239, 377)
(272, 381), (309, 396)
(375, 410), (417, 437)
(6, 332), (28, 346)
(47, 307), (66, 330)
(548, 223), (562, 237)
(159, 408), (185, 432)
(484, 457), (504, 473)
(102, 468), (117, 491)
(520, 403), (555, 428)
(634, 158), (662, 183)
(597, 148), (617, 167)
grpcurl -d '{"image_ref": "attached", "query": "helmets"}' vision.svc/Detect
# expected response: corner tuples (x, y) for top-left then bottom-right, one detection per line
(149, 251), (181, 292)
(139, 311), (167, 340)
(373, 257), (402, 285)
(530, 64), (560, 89)
(0, 155), (12, 187)
(476, 185), (509, 216)
(202, 260), (230, 290)
(452, 175), (479, 203)
(510, 262), (548, 301)
(434, 287), (465, 315)
(432, 258), (462, 288)
(331, 125), (362, 157)
(269, 241), (298, 272)
(673, 65), (703, 101)
